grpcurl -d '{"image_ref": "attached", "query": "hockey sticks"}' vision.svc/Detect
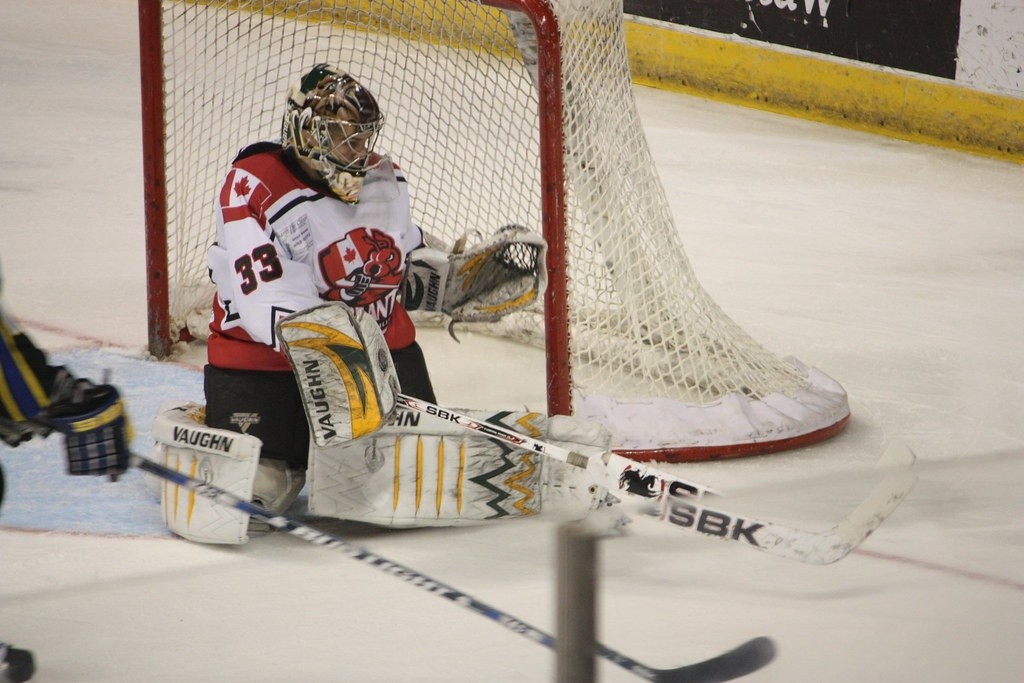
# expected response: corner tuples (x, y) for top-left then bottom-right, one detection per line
(395, 395), (920, 565)
(128, 454), (778, 683)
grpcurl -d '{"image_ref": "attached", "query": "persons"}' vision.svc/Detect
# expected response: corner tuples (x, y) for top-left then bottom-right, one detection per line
(203, 62), (551, 535)
(0, 306), (137, 683)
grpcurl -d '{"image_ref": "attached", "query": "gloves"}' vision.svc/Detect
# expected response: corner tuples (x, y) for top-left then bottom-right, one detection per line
(0, 337), (132, 480)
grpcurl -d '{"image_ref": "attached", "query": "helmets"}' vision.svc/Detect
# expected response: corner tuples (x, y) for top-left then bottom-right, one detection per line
(282, 63), (385, 206)
